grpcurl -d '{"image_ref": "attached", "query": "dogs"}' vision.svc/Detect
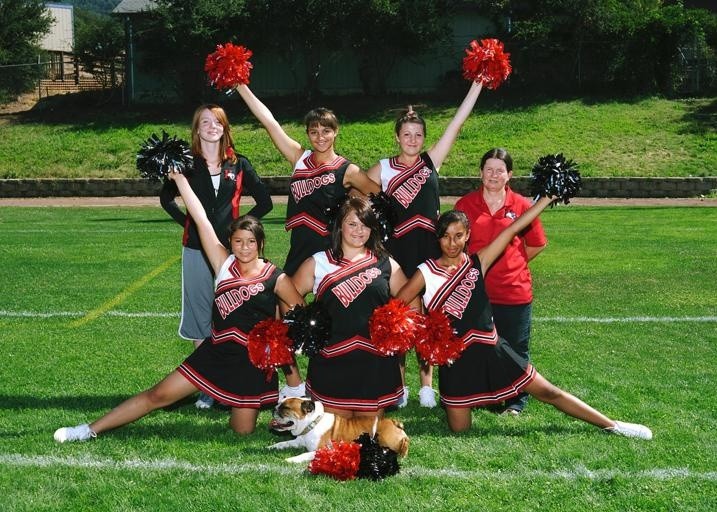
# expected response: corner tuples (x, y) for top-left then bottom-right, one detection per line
(264, 398), (409, 464)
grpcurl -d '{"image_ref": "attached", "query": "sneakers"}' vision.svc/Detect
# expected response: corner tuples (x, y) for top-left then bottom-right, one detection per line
(610, 420), (654, 441)
(275, 382), (307, 404)
(54, 423), (91, 442)
(195, 392), (216, 410)
(397, 385), (410, 408)
(419, 386), (439, 409)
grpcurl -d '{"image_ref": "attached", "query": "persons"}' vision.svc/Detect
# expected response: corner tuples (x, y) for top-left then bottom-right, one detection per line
(278, 194), (423, 421)
(159, 101), (271, 409)
(346, 77), (484, 409)
(454, 146), (548, 418)
(386, 191), (652, 437)
(53, 162), (308, 444)
(232, 80), (382, 280)
